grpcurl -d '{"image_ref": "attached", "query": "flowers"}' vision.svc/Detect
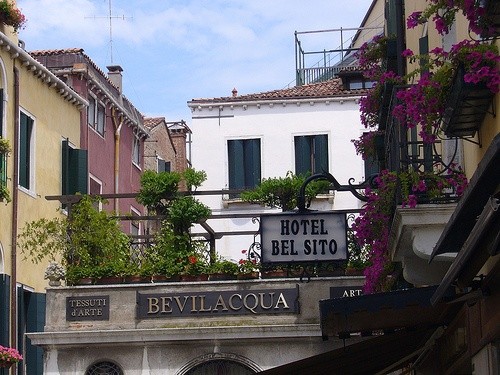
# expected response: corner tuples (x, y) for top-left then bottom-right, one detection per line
(354, 0), (500, 295)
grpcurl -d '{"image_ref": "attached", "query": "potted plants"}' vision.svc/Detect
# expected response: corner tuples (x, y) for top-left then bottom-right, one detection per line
(18, 171), (375, 286)
